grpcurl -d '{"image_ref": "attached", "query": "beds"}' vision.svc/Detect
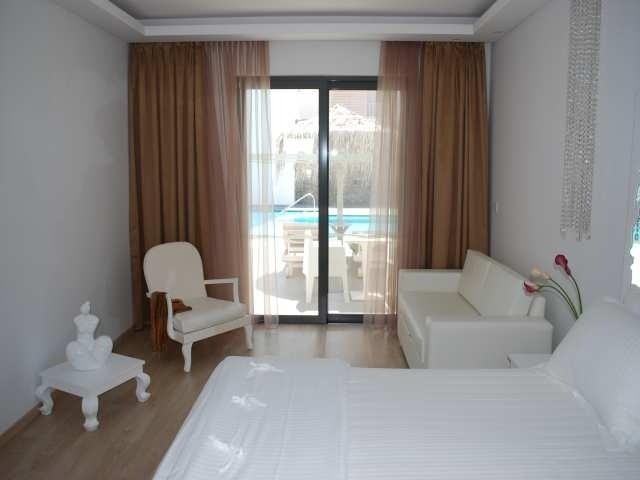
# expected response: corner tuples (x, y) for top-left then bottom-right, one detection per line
(151, 298), (639, 479)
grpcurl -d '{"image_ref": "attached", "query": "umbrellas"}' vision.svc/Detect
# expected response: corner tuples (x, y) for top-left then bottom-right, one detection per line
(277, 102), (394, 236)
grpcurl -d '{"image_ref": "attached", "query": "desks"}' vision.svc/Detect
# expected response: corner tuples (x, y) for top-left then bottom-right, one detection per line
(33, 354), (153, 433)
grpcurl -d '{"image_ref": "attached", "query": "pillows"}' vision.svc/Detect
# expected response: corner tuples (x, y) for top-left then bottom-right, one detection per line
(543, 301), (639, 449)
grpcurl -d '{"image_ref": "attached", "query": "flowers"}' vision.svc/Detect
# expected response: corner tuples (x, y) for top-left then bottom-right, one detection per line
(523, 254), (584, 320)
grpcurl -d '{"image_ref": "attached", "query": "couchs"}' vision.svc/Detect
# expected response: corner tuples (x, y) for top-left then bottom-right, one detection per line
(396, 249), (555, 369)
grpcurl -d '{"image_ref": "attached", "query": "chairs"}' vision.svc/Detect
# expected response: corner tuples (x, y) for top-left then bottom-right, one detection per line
(142, 241), (253, 373)
(281, 222), (394, 305)
